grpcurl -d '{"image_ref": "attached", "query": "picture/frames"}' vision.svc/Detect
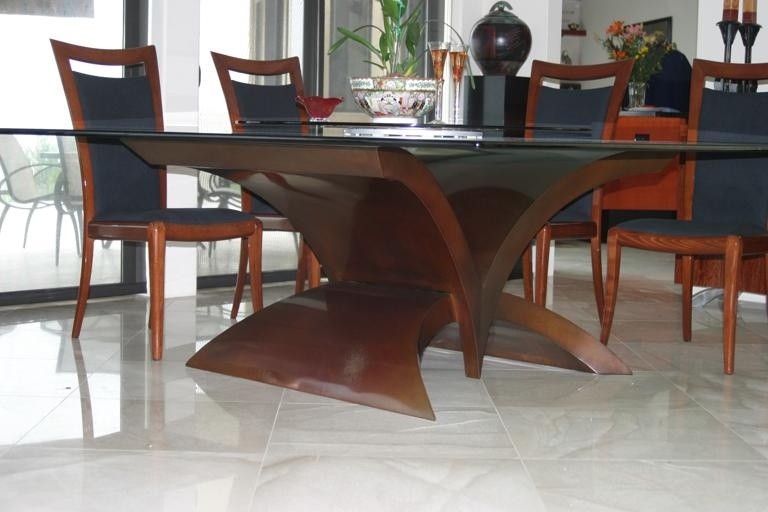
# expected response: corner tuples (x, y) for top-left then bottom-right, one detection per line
(623, 17), (672, 47)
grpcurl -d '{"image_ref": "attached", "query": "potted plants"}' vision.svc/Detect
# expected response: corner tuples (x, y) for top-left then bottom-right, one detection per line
(327, 0), (475, 125)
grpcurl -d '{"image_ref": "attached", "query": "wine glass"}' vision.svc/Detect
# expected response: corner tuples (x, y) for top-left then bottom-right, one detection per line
(445, 41), (470, 128)
(428, 39), (447, 125)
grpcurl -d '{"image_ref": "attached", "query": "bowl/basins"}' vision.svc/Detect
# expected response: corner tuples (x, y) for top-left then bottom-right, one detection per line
(295, 95), (344, 123)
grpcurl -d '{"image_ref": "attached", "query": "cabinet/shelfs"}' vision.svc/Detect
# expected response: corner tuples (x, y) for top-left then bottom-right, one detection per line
(600, 106), (688, 244)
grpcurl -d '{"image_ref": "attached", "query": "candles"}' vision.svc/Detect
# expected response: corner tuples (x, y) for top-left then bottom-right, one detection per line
(722, 0), (740, 22)
(742, 0), (757, 24)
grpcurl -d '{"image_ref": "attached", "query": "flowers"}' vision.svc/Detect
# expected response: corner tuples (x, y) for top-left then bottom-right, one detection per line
(602, 20), (677, 82)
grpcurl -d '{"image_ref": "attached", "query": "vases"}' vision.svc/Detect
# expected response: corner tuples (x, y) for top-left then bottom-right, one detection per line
(627, 82), (647, 108)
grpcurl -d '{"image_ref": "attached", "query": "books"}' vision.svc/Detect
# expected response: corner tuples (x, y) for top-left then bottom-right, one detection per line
(321, 125), (484, 139)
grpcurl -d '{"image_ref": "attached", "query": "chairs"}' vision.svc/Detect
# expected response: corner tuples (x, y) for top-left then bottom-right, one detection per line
(54, 136), (85, 267)
(522, 59), (636, 326)
(598, 58), (768, 375)
(210, 50), (320, 318)
(197, 168), (300, 255)
(51, 38), (263, 363)
(0, 134), (83, 256)
